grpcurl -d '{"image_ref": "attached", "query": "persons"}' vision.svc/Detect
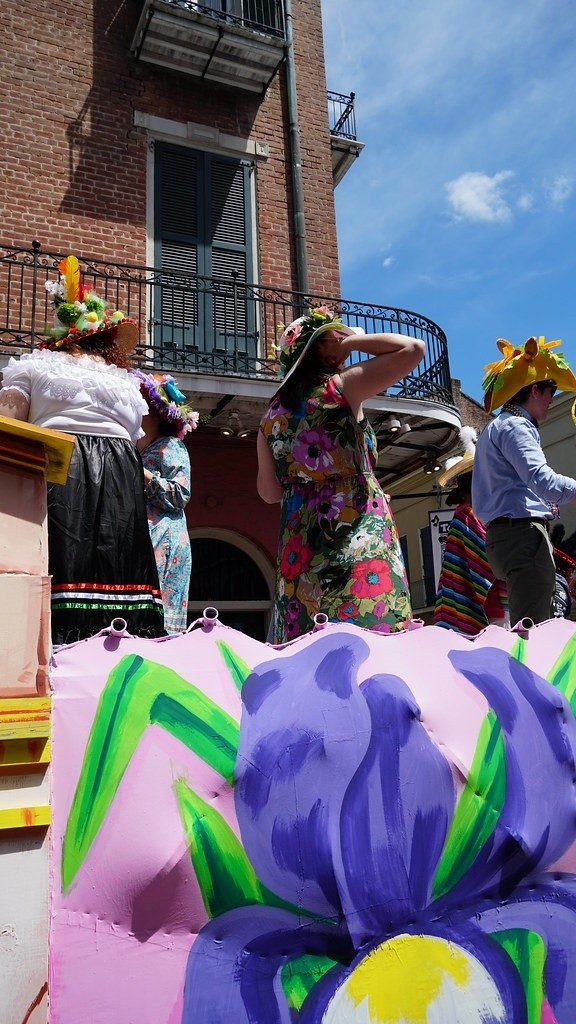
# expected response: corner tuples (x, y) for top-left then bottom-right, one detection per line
(3, 253), (167, 638)
(256, 306), (426, 633)
(471, 334), (576, 631)
(130, 367), (199, 637)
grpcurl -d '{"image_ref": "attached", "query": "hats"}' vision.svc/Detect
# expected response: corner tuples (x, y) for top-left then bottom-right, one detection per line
(485, 349), (575, 415)
(437, 447), (475, 489)
(274, 316), (365, 394)
(128, 368), (198, 424)
(38, 298), (137, 355)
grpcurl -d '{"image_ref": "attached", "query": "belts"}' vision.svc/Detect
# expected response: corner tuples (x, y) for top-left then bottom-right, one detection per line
(487, 517), (549, 532)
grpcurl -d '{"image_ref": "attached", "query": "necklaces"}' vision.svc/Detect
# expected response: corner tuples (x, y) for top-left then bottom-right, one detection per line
(501, 403), (525, 419)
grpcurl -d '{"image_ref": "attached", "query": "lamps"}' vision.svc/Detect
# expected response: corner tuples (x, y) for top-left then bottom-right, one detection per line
(220, 409), (251, 439)
(387, 415), (412, 436)
(424, 451), (443, 475)
